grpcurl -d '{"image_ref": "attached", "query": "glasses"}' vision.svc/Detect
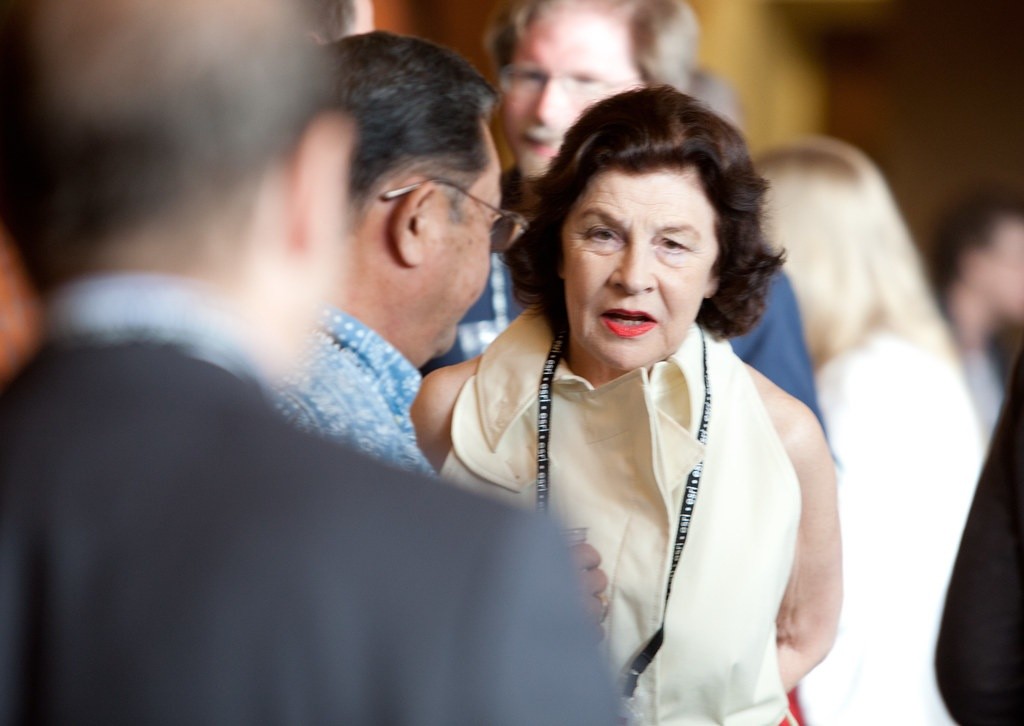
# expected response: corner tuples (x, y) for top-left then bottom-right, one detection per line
(498, 62), (648, 103)
(382, 181), (530, 252)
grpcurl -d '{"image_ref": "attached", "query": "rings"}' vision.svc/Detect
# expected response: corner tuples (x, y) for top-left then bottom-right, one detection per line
(595, 593), (608, 624)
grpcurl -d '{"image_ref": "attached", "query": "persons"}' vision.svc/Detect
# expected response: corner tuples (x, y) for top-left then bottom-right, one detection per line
(749, 140), (1024, 726)
(412, 83), (841, 726)
(272, 31), (502, 479)
(0, 0), (623, 726)
(293, 0), (374, 43)
(424, 1), (828, 458)
